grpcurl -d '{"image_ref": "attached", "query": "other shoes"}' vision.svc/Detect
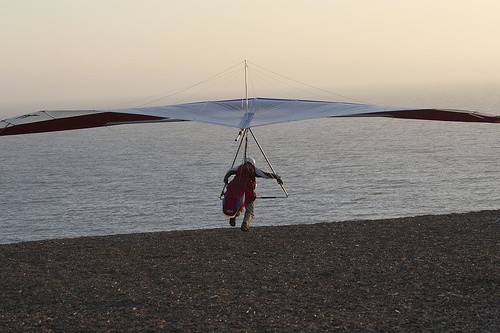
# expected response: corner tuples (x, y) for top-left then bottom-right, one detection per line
(241, 222), (252, 232)
(229, 217), (236, 227)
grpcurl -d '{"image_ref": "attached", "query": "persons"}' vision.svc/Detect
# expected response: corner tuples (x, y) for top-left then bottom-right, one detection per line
(224, 158), (284, 232)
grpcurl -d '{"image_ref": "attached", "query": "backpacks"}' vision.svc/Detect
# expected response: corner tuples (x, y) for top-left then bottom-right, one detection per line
(221, 170), (260, 217)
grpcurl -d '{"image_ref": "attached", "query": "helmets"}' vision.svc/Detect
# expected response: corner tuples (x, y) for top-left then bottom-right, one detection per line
(244, 156), (255, 165)
